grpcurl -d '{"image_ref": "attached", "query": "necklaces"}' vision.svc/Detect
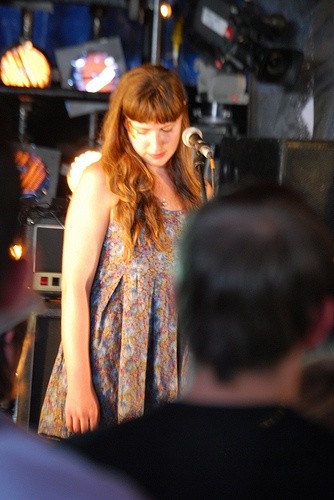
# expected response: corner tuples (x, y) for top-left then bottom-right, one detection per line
(152, 174), (172, 207)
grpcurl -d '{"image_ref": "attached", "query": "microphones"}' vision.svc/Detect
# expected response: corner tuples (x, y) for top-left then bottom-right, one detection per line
(182, 127), (214, 160)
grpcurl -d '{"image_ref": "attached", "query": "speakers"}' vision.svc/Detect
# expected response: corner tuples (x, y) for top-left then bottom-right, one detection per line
(278, 138), (334, 228)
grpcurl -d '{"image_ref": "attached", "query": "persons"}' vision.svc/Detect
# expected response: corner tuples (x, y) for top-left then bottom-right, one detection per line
(0, 80), (334, 500)
(36, 64), (220, 443)
